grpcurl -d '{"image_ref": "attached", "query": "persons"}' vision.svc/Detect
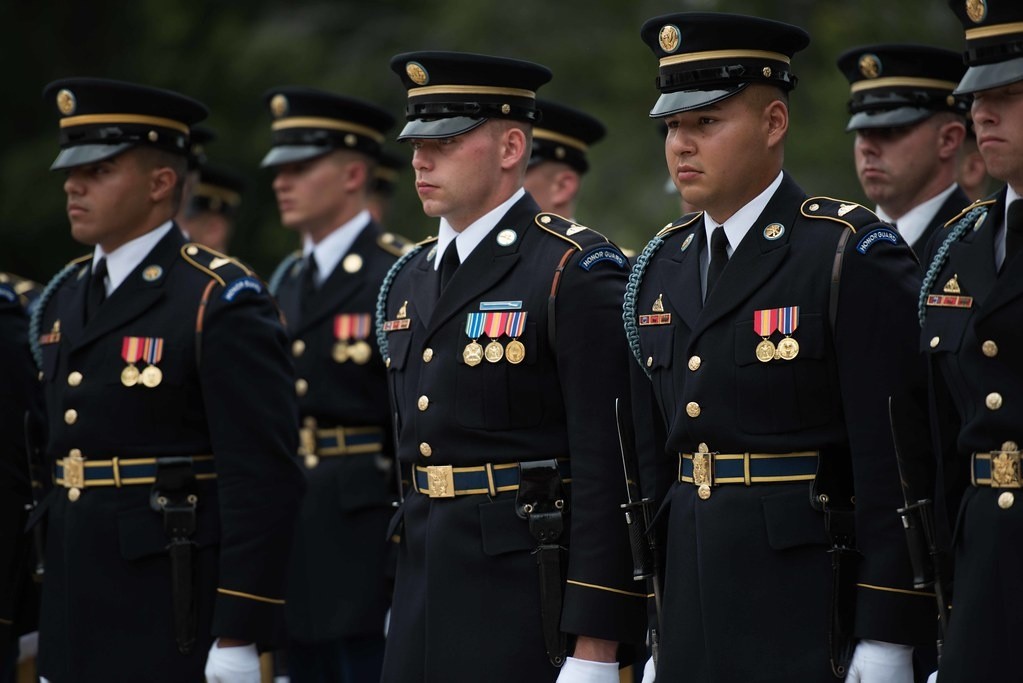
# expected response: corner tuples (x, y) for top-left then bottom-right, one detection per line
(836, 42), (987, 266)
(906, 0), (1023, 682)
(520, 98), (608, 222)
(369, 50), (629, 682)
(250, 86), (420, 683)
(618, 10), (928, 681)
(0, 75), (297, 683)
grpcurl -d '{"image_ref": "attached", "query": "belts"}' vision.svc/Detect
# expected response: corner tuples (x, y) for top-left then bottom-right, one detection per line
(409, 461), (570, 500)
(297, 425), (393, 461)
(50, 453), (223, 490)
(677, 450), (821, 485)
(968, 451), (1023, 489)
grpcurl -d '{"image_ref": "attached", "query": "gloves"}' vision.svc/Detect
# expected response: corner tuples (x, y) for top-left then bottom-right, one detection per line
(845, 641), (914, 683)
(555, 657), (620, 683)
(205, 639), (261, 683)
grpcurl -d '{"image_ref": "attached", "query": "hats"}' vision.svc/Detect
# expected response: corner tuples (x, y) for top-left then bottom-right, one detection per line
(834, 46), (974, 134)
(640, 13), (812, 119)
(180, 134), (253, 208)
(43, 75), (210, 171)
(388, 49), (551, 142)
(949, 0), (1023, 96)
(252, 88), (389, 172)
(518, 96), (609, 173)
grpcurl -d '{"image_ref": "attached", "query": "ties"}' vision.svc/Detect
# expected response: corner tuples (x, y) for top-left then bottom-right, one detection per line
(703, 227), (732, 304)
(1001, 200), (1023, 274)
(437, 238), (461, 298)
(286, 254), (319, 314)
(85, 259), (110, 323)
